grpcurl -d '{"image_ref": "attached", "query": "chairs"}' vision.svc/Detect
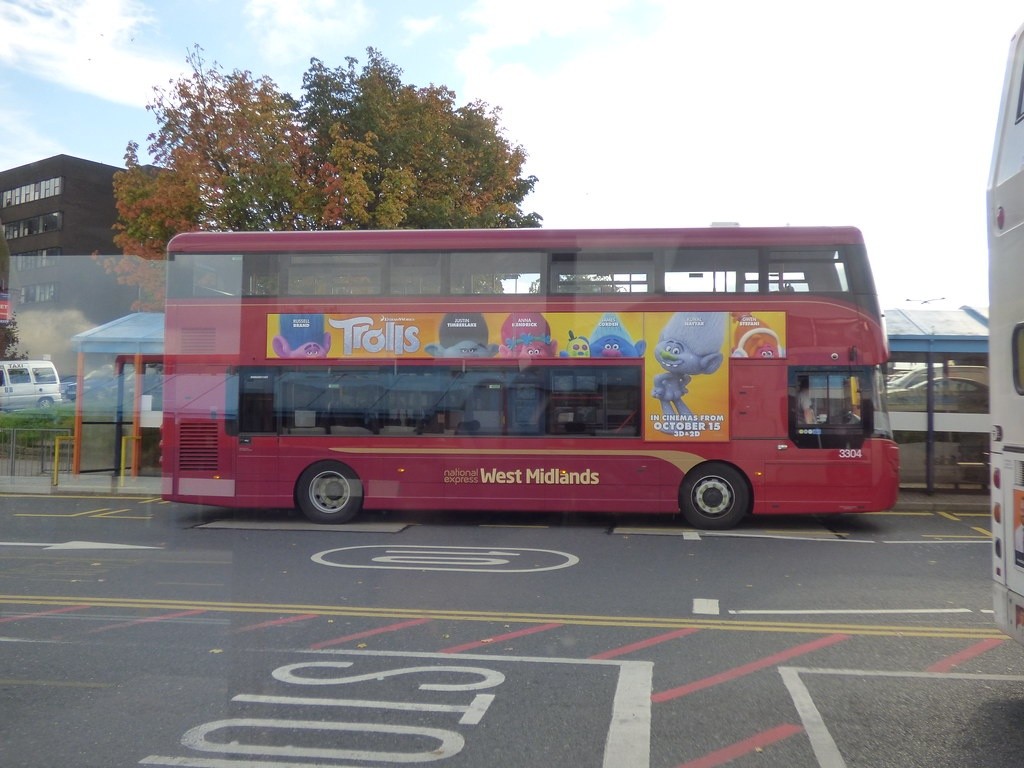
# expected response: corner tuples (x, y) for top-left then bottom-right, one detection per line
(785, 281), (794, 292)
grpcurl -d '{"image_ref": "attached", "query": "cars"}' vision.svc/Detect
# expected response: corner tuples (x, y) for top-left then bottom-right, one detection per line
(57, 366), (157, 405)
(876, 363), (988, 412)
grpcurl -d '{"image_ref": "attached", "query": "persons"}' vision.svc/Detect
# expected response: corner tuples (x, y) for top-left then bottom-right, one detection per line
(797, 380), (817, 423)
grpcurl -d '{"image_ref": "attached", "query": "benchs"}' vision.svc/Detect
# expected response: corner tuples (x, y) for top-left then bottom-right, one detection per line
(954, 461), (985, 489)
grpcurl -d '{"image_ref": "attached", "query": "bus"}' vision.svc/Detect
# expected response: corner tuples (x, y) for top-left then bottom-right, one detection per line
(985, 25), (1023, 643)
(158, 226), (900, 530)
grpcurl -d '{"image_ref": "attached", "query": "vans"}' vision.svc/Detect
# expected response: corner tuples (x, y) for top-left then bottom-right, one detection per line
(0, 360), (64, 410)
